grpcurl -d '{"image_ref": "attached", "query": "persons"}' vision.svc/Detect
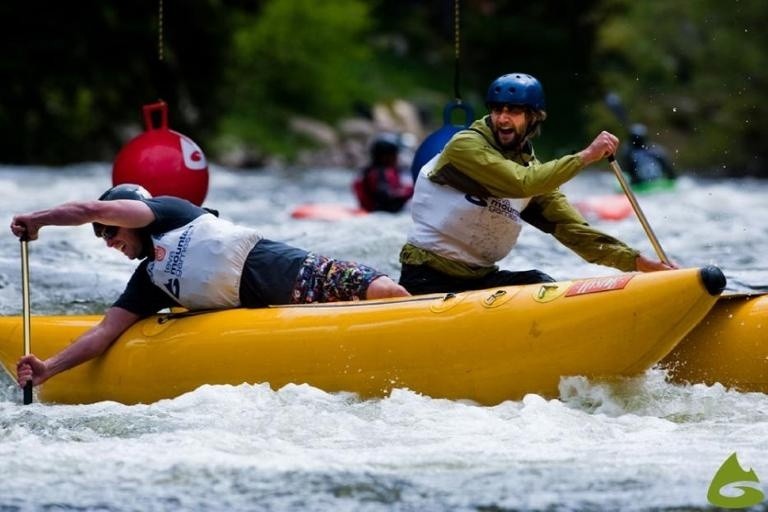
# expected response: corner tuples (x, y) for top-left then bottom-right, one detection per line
(621, 122), (677, 182)
(398, 73), (680, 296)
(11, 182), (414, 386)
(352, 132), (413, 213)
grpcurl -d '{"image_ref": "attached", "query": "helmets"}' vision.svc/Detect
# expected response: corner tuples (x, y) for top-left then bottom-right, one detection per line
(485, 72), (545, 110)
(92, 183), (153, 237)
(368, 134), (399, 154)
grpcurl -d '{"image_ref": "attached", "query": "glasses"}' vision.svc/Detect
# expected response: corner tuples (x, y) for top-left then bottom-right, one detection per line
(492, 103), (530, 112)
(101, 227), (120, 240)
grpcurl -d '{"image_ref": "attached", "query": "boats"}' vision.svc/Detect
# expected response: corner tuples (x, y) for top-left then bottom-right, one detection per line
(1, 267), (768, 404)
(290, 195), (633, 221)
(614, 172), (678, 193)
